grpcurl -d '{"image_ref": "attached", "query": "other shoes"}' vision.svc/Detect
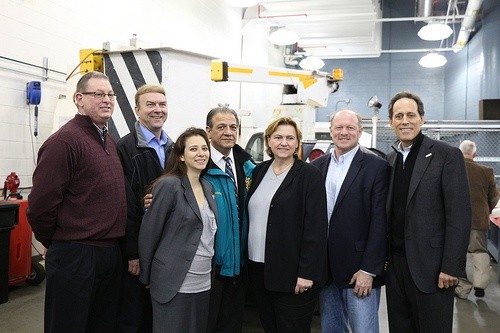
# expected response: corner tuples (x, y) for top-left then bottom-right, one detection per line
(474, 287), (485, 297)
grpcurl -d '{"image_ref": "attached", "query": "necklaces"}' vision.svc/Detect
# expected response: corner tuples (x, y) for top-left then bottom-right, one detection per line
(270, 159), (294, 175)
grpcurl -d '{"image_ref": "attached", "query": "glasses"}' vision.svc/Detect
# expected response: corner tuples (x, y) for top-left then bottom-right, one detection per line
(82, 91), (118, 98)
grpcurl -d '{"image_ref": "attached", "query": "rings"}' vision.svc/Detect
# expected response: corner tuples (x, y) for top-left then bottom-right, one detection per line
(303, 288), (308, 290)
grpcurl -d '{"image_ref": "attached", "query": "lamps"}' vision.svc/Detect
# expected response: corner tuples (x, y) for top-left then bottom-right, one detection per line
(367, 96), (383, 120)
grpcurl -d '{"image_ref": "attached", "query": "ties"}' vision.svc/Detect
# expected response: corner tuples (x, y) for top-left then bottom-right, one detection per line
(223, 157), (240, 214)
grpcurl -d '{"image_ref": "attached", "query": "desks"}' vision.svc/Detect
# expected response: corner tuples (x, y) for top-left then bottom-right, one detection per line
(0, 202), (20, 304)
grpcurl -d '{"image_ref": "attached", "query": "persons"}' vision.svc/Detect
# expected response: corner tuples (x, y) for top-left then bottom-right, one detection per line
(384, 93), (472, 333)
(240, 117), (329, 333)
(308, 109), (392, 333)
(454, 140), (498, 299)
(137, 127), (218, 333)
(115, 85), (176, 333)
(26, 72), (127, 333)
(143, 106), (256, 333)
